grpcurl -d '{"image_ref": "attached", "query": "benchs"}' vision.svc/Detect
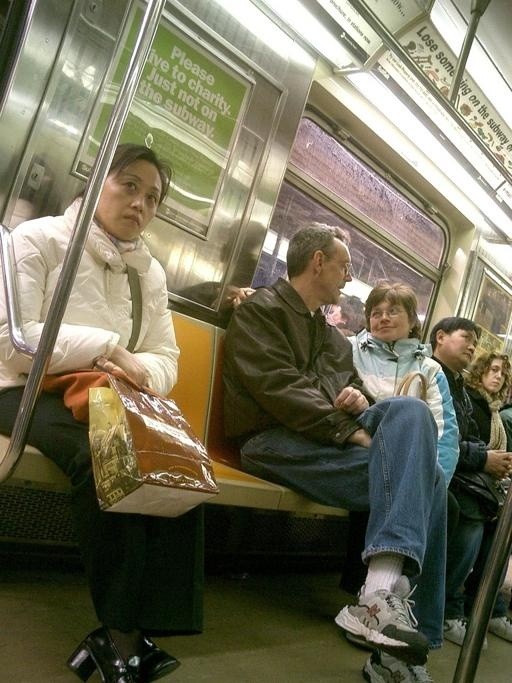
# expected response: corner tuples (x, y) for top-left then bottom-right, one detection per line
(1, 308), (351, 518)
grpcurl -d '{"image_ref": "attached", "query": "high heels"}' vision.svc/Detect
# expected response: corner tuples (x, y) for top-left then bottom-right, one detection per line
(65, 625), (179, 682)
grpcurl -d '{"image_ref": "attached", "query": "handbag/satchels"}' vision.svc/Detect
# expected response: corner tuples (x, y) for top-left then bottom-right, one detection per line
(40, 365), (175, 426)
(449, 470), (512, 521)
(88, 373), (220, 520)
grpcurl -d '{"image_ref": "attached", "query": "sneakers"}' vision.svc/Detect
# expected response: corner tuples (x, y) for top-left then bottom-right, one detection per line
(362, 647), (433, 683)
(444, 618), (489, 650)
(489, 614), (512, 642)
(333, 575), (431, 665)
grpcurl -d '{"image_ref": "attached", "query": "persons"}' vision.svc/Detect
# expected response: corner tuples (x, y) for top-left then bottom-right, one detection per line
(232, 280), (461, 652)
(429, 317), (512, 651)
(0, 153), (60, 230)
(499, 404), (512, 452)
(223, 221), (448, 683)
(0, 143), (204, 683)
(462, 351), (512, 452)
(323, 296), (368, 337)
